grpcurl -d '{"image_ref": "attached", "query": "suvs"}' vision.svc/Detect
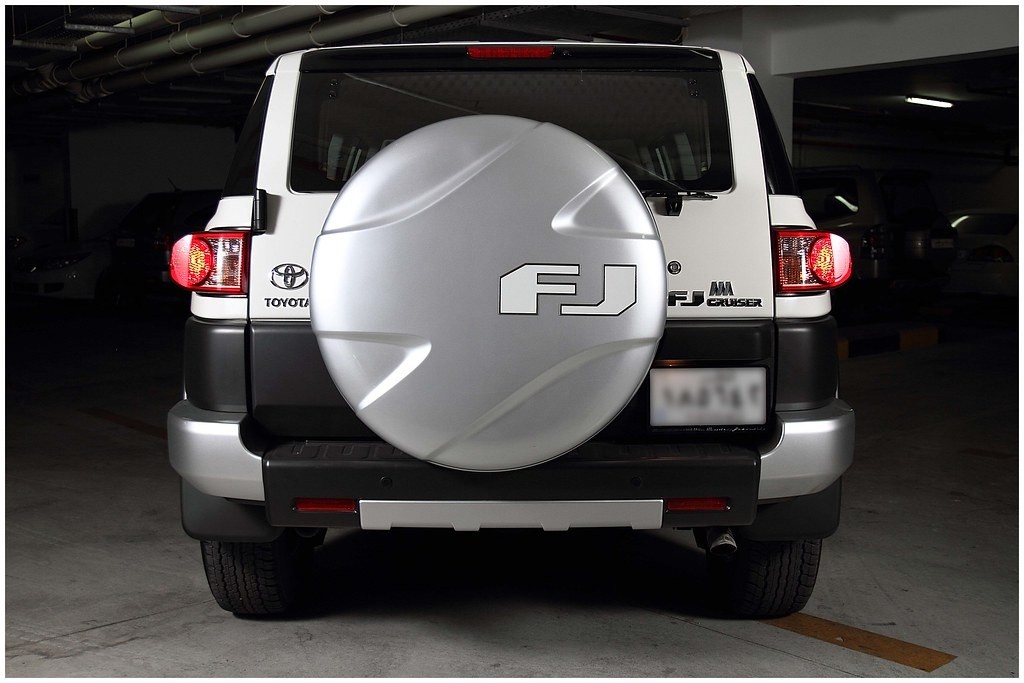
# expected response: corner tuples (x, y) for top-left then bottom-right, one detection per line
(793, 164), (957, 313)
(165, 42), (861, 622)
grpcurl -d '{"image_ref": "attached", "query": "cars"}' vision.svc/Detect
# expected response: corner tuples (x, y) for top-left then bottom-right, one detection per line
(941, 210), (1018, 325)
(8, 189), (229, 319)
